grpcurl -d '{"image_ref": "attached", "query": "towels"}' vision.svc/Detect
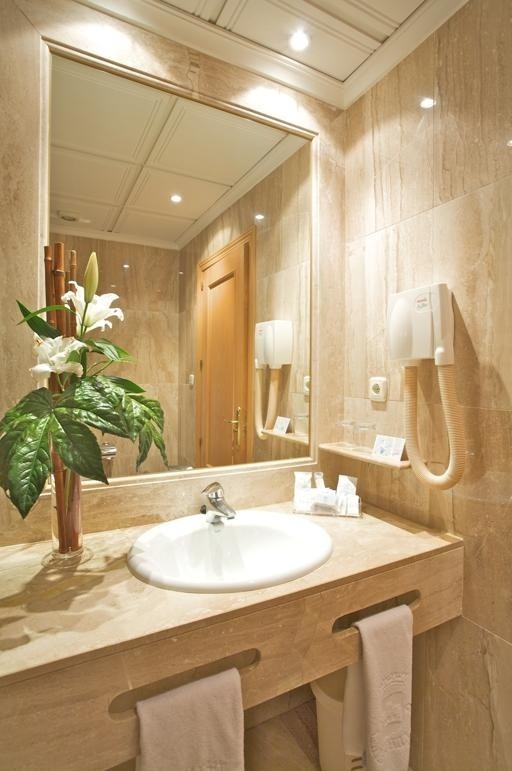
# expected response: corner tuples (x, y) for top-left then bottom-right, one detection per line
(132, 667), (250, 771)
(341, 600), (415, 771)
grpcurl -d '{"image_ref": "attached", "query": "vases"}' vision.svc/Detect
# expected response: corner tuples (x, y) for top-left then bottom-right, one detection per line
(49, 453), (83, 560)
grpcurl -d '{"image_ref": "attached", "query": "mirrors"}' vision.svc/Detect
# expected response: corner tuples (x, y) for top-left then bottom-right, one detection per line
(39, 39), (322, 492)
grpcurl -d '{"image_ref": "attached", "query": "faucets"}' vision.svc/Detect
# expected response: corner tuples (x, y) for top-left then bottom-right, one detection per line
(200, 482), (236, 523)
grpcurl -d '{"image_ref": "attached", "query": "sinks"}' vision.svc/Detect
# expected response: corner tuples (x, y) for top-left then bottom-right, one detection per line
(127, 508), (333, 592)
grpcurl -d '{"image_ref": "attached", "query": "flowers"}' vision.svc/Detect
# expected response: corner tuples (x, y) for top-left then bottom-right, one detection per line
(1, 250), (169, 520)
